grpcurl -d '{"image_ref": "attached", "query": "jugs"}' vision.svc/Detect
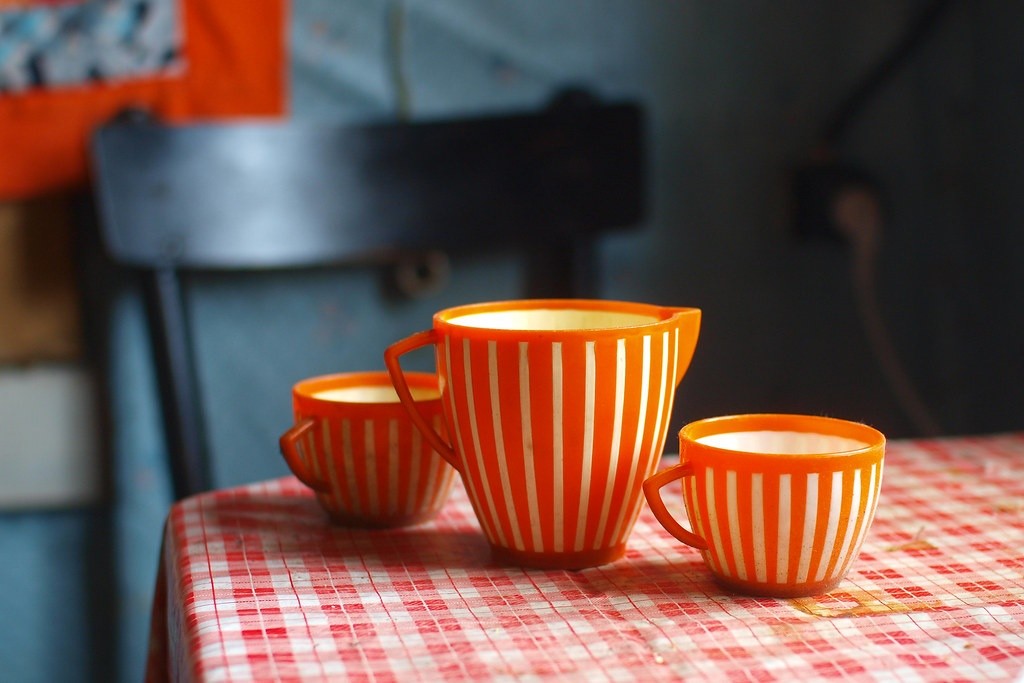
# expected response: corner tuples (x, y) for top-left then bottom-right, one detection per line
(384, 298), (701, 570)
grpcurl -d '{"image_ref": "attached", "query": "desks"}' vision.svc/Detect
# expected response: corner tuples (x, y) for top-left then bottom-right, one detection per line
(144, 434), (1024, 683)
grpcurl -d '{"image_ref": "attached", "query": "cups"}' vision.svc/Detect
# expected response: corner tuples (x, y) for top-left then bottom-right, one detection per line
(279, 370), (455, 530)
(642, 413), (887, 597)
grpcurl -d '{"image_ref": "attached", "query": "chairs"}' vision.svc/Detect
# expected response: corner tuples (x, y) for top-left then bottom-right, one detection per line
(96, 79), (647, 498)
